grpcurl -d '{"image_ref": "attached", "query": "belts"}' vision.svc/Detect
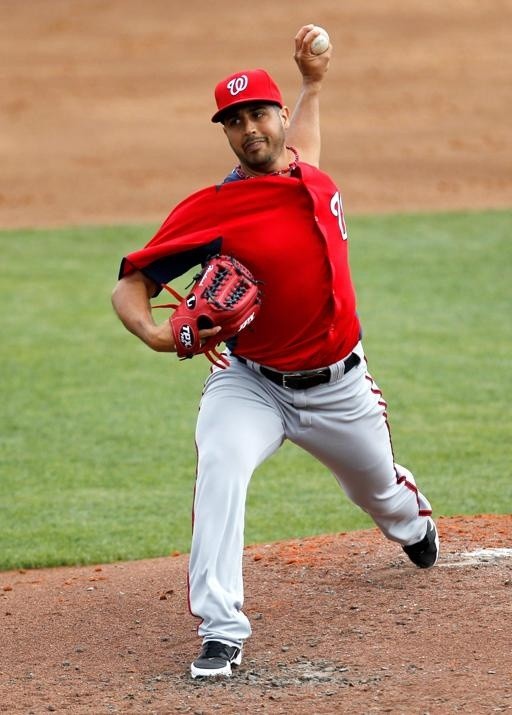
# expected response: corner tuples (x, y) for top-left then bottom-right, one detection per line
(229, 353), (360, 390)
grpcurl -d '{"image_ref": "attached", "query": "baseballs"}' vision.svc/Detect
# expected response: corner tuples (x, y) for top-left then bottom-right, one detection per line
(311, 27), (330, 54)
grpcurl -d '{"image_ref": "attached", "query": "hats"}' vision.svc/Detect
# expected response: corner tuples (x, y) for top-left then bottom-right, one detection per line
(211, 70), (283, 123)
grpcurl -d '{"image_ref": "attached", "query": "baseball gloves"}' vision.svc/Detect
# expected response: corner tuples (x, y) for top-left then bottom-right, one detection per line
(170, 254), (261, 357)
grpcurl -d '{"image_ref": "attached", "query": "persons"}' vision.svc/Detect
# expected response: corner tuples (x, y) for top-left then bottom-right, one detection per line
(111, 22), (439, 679)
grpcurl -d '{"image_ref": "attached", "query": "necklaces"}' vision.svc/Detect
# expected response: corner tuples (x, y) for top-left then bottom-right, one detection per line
(235, 144), (301, 181)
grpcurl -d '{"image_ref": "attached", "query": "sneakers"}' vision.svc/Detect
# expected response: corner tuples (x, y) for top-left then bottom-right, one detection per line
(401, 516), (439, 569)
(190, 641), (243, 679)
